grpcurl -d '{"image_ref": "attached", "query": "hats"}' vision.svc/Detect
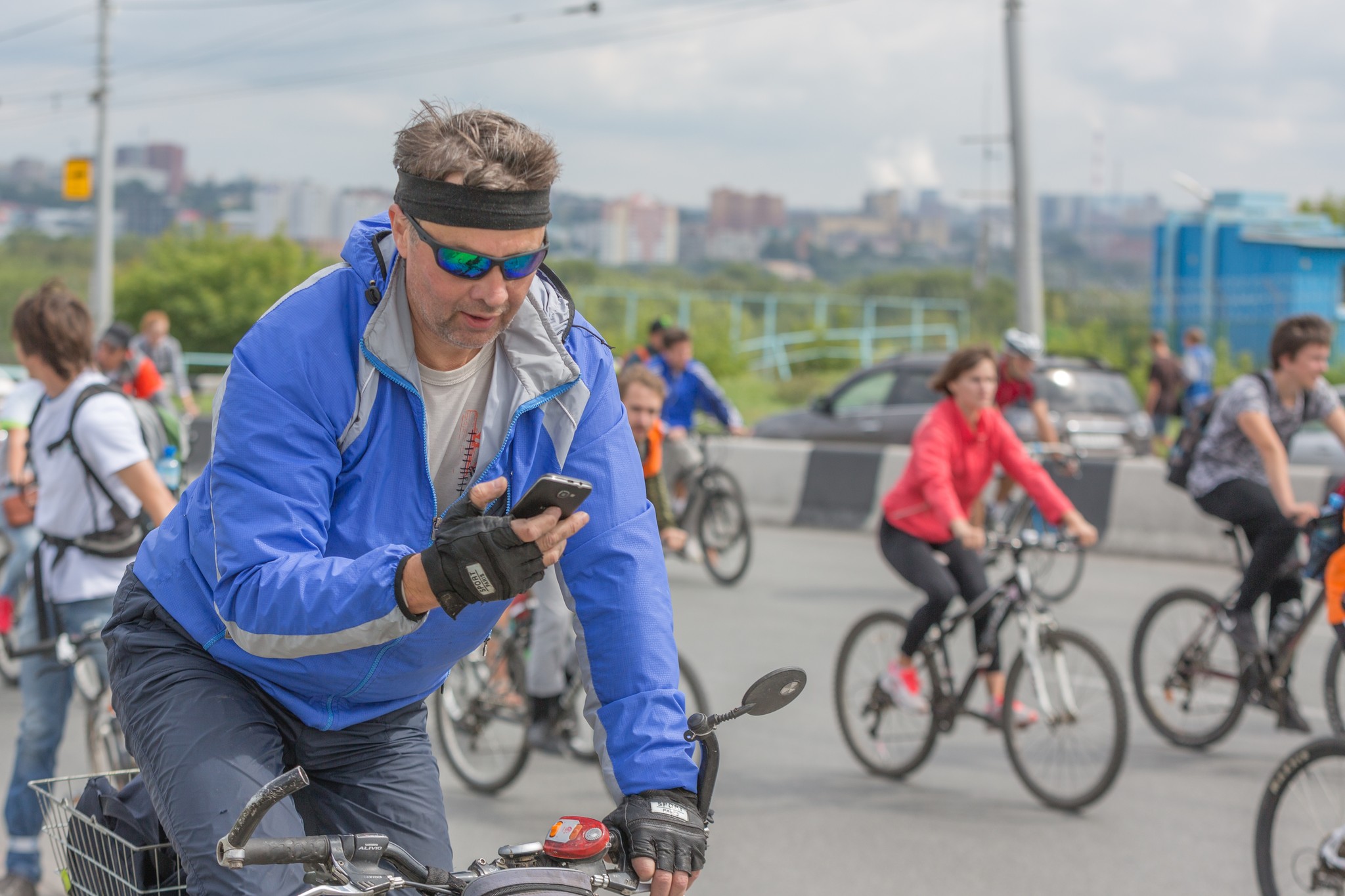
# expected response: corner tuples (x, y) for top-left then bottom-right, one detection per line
(650, 316), (674, 331)
(97, 321), (135, 351)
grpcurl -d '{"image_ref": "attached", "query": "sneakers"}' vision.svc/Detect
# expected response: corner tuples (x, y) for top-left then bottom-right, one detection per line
(881, 658), (931, 713)
(984, 695), (1035, 730)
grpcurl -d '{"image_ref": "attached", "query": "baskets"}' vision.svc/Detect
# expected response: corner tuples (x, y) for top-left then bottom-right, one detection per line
(28, 768), (187, 895)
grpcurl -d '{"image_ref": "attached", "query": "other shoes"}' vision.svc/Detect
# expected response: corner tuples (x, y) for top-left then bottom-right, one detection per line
(1239, 659), (1295, 710)
(1221, 606), (1264, 655)
(0, 876), (32, 895)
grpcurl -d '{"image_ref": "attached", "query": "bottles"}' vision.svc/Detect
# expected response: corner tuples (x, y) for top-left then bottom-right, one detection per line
(155, 446), (182, 491)
(1303, 494), (1341, 578)
(1267, 598), (1302, 652)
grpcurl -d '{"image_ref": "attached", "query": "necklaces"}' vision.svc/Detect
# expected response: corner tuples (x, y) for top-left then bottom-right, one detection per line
(876, 343), (1100, 729)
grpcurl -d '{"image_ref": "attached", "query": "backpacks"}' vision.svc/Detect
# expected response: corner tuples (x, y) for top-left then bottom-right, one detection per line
(1166, 366), (1315, 489)
(27, 383), (175, 559)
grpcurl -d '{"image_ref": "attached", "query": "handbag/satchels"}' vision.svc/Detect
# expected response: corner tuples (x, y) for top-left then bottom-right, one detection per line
(4, 494), (36, 525)
(65, 774), (187, 896)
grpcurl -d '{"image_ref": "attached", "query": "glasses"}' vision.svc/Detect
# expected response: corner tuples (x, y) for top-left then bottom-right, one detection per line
(396, 204), (551, 284)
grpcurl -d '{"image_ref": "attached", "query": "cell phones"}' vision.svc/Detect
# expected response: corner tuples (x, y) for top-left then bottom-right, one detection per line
(508, 470), (593, 523)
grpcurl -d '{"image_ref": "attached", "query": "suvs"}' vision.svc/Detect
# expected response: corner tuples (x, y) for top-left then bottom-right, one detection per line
(755, 352), (1162, 459)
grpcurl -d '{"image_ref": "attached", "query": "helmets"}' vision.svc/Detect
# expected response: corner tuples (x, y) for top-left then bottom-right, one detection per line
(1002, 327), (1044, 359)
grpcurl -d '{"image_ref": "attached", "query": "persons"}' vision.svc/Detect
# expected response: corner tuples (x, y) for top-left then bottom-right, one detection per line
(1185, 312), (1345, 735)
(2, 276), (184, 896)
(101, 101), (710, 895)
(1142, 330), (1193, 455)
(1179, 327), (1218, 418)
(3, 300), (748, 684)
(976, 325), (1080, 567)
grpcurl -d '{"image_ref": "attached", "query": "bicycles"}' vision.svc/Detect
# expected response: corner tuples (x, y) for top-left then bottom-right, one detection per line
(44, 660), (806, 896)
(1130, 472), (1345, 753)
(441, 538), (710, 815)
(1249, 726), (1343, 896)
(965, 445), (1087, 599)
(832, 523), (1135, 812)
(670, 420), (751, 586)
